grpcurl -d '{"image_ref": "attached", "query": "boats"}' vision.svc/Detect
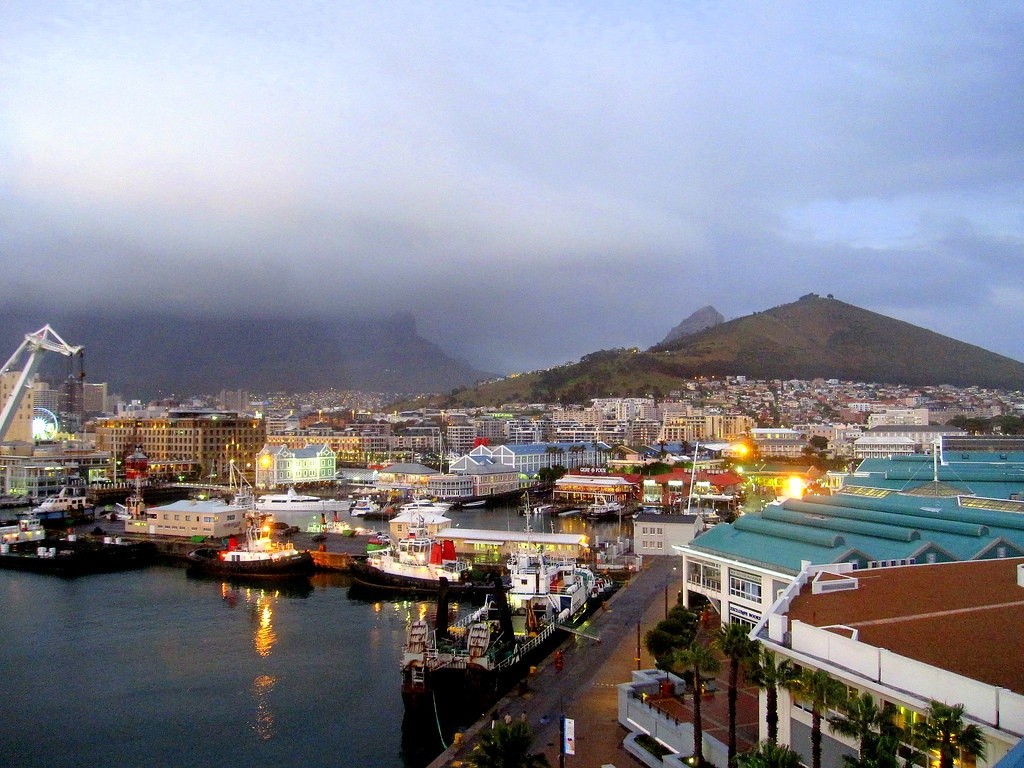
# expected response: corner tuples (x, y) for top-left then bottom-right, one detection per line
(252, 488), (352, 511)
(184, 547), (314, 583)
(345, 528), (612, 693)
(349, 497), (487, 517)
(33, 487), (94, 526)
(0, 516), (156, 567)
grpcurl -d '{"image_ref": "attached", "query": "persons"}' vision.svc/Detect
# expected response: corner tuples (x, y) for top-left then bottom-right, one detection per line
(565, 738), (573, 751)
(701, 609), (710, 629)
(503, 711), (512, 727)
(489, 709), (499, 730)
(556, 650), (563, 673)
(539, 715), (550, 730)
(450, 730), (481, 768)
(521, 711), (528, 722)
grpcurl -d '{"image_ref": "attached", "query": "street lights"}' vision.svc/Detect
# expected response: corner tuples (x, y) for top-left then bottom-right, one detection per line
(665, 566), (677, 619)
